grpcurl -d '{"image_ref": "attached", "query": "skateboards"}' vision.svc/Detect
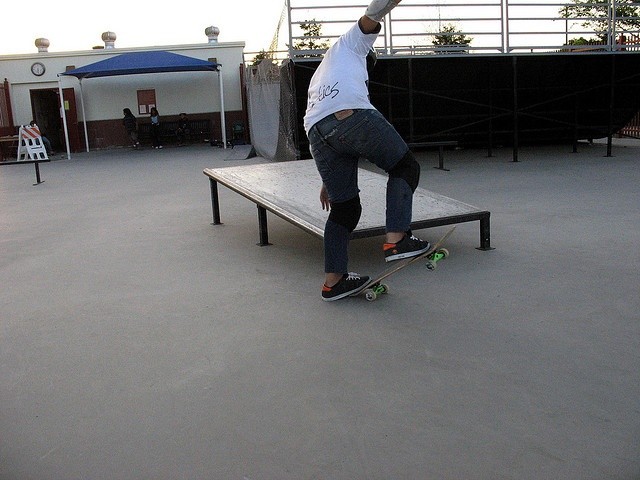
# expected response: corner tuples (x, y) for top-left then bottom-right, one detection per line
(348, 223), (458, 302)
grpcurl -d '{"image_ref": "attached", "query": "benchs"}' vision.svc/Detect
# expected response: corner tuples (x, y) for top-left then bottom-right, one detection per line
(139, 119), (211, 149)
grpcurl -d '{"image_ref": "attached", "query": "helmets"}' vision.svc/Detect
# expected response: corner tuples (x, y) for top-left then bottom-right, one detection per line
(366, 47), (377, 71)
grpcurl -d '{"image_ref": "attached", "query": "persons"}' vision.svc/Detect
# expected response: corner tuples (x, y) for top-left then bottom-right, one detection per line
(31, 120), (54, 158)
(175, 113), (192, 147)
(121, 108), (141, 150)
(149, 107), (163, 149)
(302, 1), (431, 302)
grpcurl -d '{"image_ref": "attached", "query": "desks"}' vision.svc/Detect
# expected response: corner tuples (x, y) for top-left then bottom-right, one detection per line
(201, 156), (496, 252)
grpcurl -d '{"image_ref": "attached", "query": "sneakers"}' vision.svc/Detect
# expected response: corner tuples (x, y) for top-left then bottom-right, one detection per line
(321, 271), (372, 302)
(383, 233), (431, 263)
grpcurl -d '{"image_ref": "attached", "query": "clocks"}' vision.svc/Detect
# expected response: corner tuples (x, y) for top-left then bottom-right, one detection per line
(30, 62), (45, 76)
